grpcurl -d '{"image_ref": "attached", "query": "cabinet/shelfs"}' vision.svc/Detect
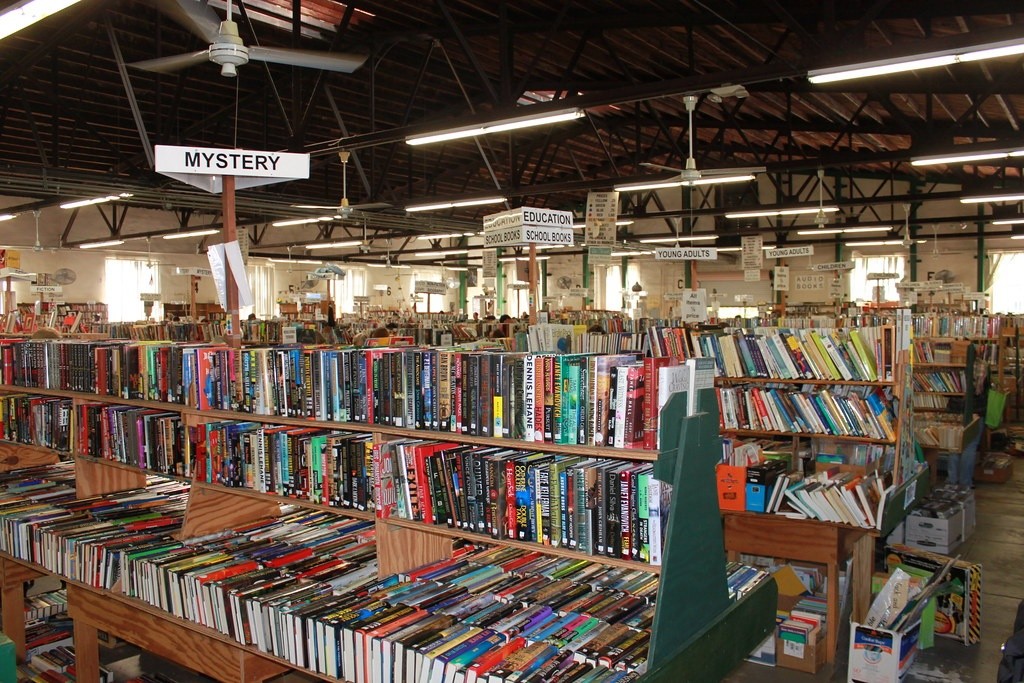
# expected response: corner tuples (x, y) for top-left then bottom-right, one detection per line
(0, 303), (1024, 683)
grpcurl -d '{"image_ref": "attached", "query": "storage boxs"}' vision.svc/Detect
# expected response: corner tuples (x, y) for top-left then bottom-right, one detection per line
(746, 459), (788, 511)
(962, 498), (976, 542)
(775, 634), (826, 673)
(743, 626), (779, 666)
(885, 521), (904, 548)
(904, 509), (963, 555)
(973, 463), (1013, 482)
(715, 462), (752, 511)
(847, 613), (921, 683)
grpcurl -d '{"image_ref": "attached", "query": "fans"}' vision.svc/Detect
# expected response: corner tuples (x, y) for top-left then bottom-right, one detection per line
(53, 268), (76, 284)
(127, 0), (365, 77)
(142, 237), (175, 268)
(337, 212), (391, 253)
(639, 98), (765, 183)
(920, 225), (960, 258)
(6, 210), (72, 253)
(774, 171), (856, 226)
(893, 203), (933, 248)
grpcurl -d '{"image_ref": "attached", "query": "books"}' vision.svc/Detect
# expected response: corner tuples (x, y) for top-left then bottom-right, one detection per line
(216, 347), (446, 525)
(896, 308), (1024, 446)
(102, 321), (221, 341)
(338, 305), (467, 321)
(209, 313), (226, 320)
(284, 304), (315, 320)
(438, 352), (715, 566)
(0, 484), (190, 616)
(189, 517), (572, 683)
(0, 343), (216, 482)
(240, 321), (515, 345)
(726, 553), (845, 667)
(717, 387), (897, 529)
(573, 566), (659, 683)
(527, 308), (893, 381)
(21, 303), (108, 332)
(24, 616), (182, 683)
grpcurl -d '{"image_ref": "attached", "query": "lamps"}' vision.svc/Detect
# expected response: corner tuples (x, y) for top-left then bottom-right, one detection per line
(0, 0), (1024, 269)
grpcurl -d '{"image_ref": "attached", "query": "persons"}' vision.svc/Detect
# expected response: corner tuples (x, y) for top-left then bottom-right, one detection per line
(387, 322), (397, 330)
(172, 316), (180, 321)
(372, 327), (389, 338)
(247, 313), (256, 320)
(719, 322), (730, 329)
(201, 319), (209, 323)
(353, 333), (369, 346)
(587, 325), (606, 334)
(92, 314), (99, 322)
(473, 312), (478, 321)
(483, 315), (495, 320)
(499, 315), (512, 322)
